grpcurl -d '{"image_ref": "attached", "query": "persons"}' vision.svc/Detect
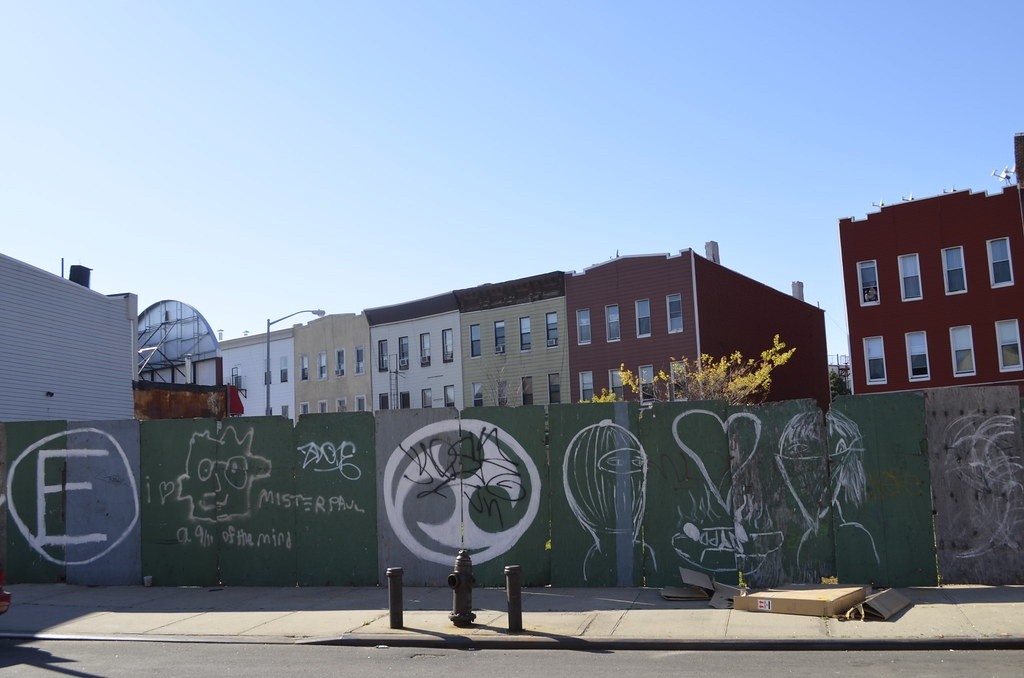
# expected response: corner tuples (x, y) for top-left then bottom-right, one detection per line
(865, 288), (877, 301)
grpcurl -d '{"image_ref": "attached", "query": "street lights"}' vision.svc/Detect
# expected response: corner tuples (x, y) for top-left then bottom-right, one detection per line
(266, 309), (325, 416)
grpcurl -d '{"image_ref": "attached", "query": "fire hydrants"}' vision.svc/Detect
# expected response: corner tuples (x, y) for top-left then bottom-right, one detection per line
(448, 549), (477, 628)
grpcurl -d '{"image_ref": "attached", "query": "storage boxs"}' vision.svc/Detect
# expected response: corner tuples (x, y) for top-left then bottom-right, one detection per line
(731, 582), (872, 616)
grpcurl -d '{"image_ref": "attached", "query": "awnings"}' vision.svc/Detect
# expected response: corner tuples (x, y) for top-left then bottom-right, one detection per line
(230, 385), (244, 415)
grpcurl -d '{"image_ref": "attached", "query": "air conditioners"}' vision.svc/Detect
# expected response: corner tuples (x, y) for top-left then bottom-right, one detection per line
(335, 369), (344, 376)
(547, 339), (556, 345)
(421, 356), (430, 364)
(400, 359), (408, 366)
(494, 345), (505, 354)
